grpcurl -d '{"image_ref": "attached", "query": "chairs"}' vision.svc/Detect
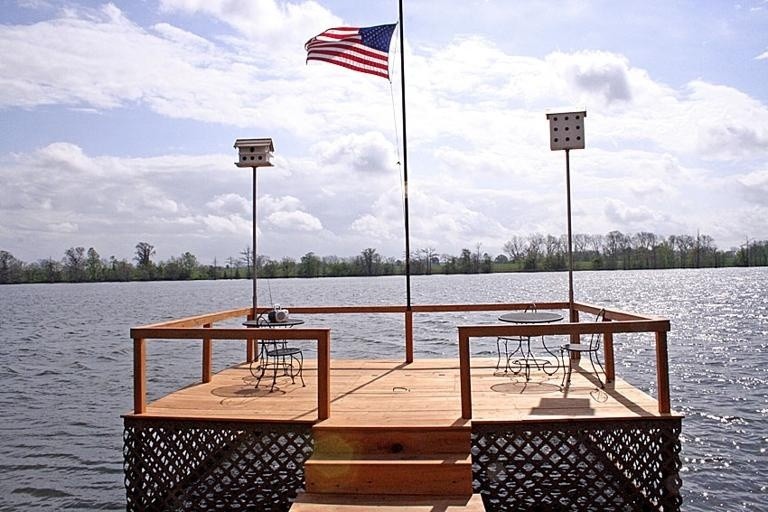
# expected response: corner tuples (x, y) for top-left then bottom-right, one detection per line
(497, 303), (541, 372)
(255, 316), (306, 393)
(560, 309), (611, 392)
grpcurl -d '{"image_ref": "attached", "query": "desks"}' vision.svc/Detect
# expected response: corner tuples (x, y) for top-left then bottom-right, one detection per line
(499, 312), (564, 376)
(242, 319), (305, 379)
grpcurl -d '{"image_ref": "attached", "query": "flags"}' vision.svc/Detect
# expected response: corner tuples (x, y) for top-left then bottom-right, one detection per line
(304, 21), (397, 80)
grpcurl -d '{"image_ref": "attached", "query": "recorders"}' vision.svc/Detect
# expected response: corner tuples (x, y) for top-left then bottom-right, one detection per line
(269, 304), (288, 323)
